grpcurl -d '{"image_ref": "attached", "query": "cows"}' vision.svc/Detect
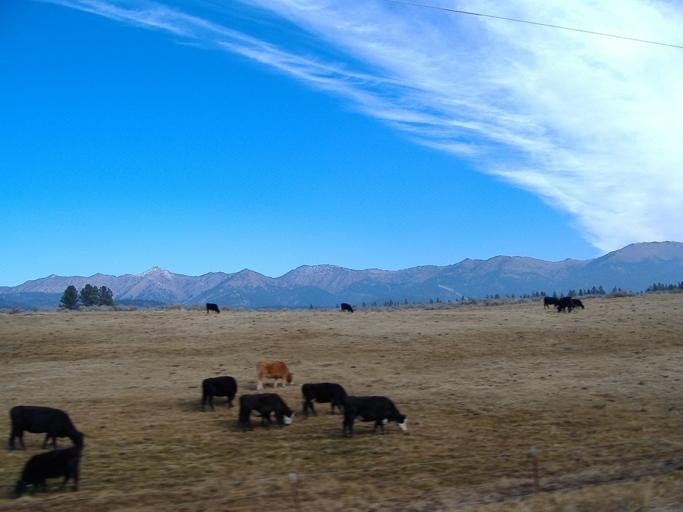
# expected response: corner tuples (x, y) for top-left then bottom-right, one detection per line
(206, 302), (221, 314)
(201, 375), (238, 411)
(340, 303), (353, 313)
(302, 382), (348, 417)
(544, 295), (585, 314)
(237, 392), (295, 432)
(14, 446), (81, 496)
(7, 404), (84, 449)
(341, 395), (408, 438)
(255, 360), (294, 390)
